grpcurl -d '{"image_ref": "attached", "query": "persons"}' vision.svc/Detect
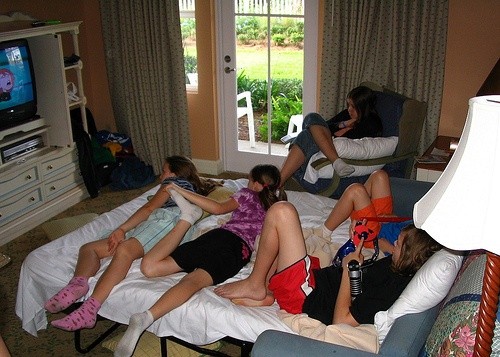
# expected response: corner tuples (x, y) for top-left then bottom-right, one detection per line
(319, 170), (416, 256)
(279, 87), (392, 187)
(114, 164), (286, 357)
(213, 201), (437, 328)
(44, 155), (204, 331)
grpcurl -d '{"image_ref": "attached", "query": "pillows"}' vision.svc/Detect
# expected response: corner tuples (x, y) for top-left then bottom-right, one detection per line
(303, 134), (399, 186)
(373, 249), (463, 340)
(42, 212), (99, 242)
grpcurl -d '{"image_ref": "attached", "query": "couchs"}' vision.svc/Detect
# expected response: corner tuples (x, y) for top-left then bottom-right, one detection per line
(290, 81), (429, 197)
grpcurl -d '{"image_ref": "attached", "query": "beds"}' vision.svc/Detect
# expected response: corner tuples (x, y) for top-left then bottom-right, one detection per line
(17, 168), (500, 357)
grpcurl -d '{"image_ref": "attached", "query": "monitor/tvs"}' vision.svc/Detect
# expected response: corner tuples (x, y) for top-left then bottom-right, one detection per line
(0, 37), (39, 131)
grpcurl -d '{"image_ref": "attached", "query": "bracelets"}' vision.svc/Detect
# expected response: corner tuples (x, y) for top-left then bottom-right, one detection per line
(341, 121), (346, 126)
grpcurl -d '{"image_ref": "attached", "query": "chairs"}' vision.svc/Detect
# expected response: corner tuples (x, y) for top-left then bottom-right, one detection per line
(187, 72), (257, 149)
(285, 114), (303, 149)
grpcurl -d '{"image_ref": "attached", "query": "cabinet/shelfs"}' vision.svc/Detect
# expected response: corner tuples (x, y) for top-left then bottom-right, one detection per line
(0, 20), (91, 247)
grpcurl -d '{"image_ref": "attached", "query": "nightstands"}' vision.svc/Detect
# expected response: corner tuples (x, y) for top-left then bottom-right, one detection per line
(413, 133), (460, 183)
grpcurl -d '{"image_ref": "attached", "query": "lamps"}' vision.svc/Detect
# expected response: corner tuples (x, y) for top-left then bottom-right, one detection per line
(409, 95), (500, 357)
